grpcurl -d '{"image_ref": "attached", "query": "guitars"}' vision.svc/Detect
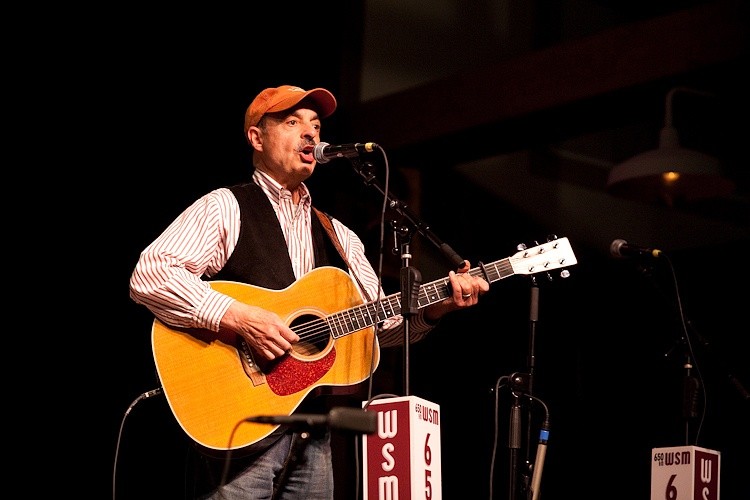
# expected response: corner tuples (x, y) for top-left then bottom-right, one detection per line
(150, 234), (579, 451)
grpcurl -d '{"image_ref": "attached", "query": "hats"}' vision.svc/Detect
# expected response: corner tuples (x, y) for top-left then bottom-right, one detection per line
(244, 85), (337, 143)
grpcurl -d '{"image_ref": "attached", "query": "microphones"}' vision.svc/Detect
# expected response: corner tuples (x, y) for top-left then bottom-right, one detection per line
(610, 238), (662, 258)
(246, 406), (376, 434)
(312, 142), (377, 164)
(529, 422), (549, 500)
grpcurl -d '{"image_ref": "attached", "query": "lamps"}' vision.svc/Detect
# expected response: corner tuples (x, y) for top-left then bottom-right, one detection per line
(607, 88), (719, 198)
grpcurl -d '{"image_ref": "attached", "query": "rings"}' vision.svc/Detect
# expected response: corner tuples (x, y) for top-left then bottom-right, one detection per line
(462, 293), (472, 298)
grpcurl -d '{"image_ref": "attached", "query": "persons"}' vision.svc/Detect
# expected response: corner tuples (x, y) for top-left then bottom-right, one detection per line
(129, 85), (489, 500)
